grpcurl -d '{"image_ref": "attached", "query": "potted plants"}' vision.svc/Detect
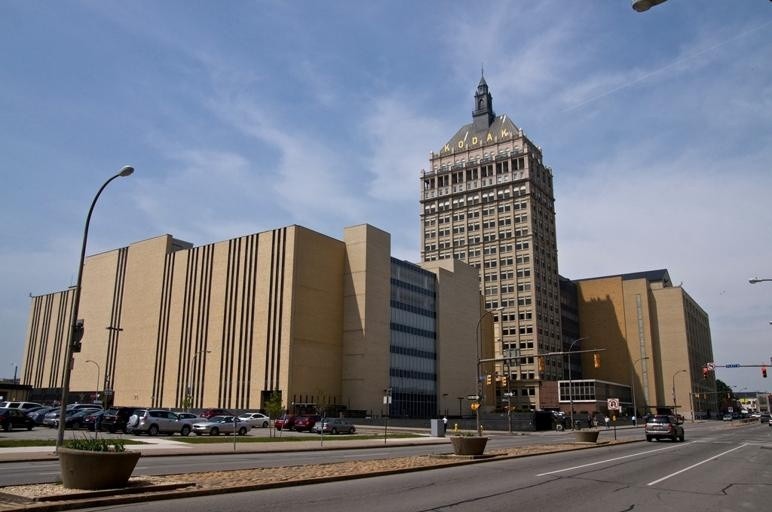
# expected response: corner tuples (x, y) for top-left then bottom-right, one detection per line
(450, 431), (489, 455)
(56, 429), (142, 489)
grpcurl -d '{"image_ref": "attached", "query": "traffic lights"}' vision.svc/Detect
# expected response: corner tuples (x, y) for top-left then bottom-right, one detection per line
(594, 352), (601, 368)
(71, 318), (84, 353)
(538, 358), (544, 372)
(502, 376), (507, 386)
(471, 403), (481, 410)
(704, 367), (709, 380)
(487, 375), (492, 385)
(762, 367), (767, 378)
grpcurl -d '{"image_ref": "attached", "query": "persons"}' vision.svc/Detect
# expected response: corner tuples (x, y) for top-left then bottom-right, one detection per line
(631, 414), (636, 426)
(604, 415), (611, 429)
(612, 415), (616, 428)
(592, 414), (598, 430)
(441, 414), (448, 434)
(587, 415), (592, 429)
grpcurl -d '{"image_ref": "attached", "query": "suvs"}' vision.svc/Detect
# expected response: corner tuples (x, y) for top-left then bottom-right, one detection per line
(644, 414), (686, 444)
(275, 414), (297, 431)
(293, 414), (324, 433)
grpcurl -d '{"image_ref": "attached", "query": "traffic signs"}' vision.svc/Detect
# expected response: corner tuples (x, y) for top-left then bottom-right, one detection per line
(726, 364), (740, 368)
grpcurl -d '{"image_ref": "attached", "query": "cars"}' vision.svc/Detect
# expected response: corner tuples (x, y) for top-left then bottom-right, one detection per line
(723, 412), (772, 427)
(0, 400), (208, 437)
(237, 412), (271, 428)
(191, 415), (252, 437)
(312, 417), (355, 435)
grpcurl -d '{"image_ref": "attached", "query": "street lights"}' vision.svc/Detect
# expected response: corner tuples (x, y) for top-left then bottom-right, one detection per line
(672, 369), (687, 422)
(187, 350), (212, 412)
(56, 162), (136, 455)
(632, 356), (650, 427)
(85, 360), (100, 403)
(475, 307), (506, 437)
(568, 336), (595, 430)
(749, 275), (772, 284)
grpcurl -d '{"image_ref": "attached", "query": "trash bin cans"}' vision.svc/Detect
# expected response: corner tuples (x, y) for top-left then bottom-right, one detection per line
(574, 420), (581, 430)
